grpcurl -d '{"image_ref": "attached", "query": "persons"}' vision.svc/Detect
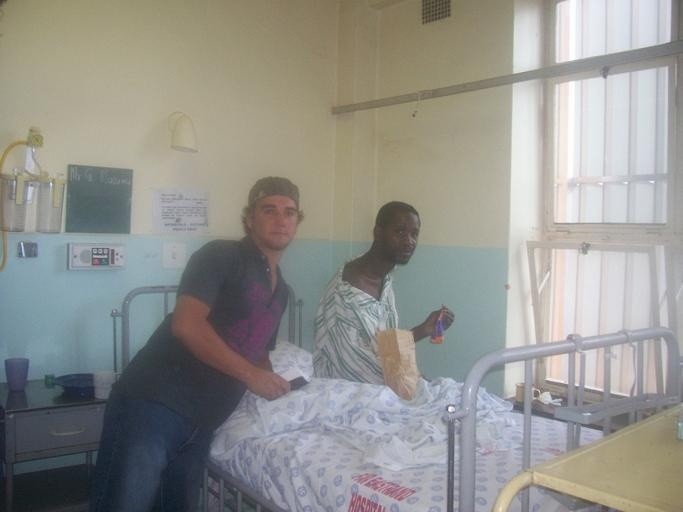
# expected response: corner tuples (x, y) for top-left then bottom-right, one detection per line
(313, 201), (455, 387)
(92, 176), (306, 512)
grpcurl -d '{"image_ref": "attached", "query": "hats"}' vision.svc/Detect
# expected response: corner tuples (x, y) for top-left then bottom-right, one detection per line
(249, 176), (299, 209)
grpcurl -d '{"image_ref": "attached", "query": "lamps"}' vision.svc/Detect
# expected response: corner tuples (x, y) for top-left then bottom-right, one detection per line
(166, 110), (200, 154)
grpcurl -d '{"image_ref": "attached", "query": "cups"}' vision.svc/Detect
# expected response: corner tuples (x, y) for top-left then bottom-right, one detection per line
(4, 358), (30, 391)
(94, 372), (114, 400)
(6, 392), (27, 409)
(0, 174), (64, 233)
(515, 383), (541, 402)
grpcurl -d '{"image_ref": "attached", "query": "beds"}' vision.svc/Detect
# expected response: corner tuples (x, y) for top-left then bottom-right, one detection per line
(111, 280), (680, 512)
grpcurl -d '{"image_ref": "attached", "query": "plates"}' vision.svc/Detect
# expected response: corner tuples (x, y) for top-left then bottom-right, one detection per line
(53, 374), (96, 393)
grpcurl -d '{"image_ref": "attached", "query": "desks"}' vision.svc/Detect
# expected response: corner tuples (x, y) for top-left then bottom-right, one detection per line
(491, 403), (681, 512)
(0, 373), (109, 512)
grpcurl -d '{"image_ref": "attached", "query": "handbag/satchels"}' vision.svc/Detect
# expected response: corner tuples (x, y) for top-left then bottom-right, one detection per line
(377, 327), (417, 400)
